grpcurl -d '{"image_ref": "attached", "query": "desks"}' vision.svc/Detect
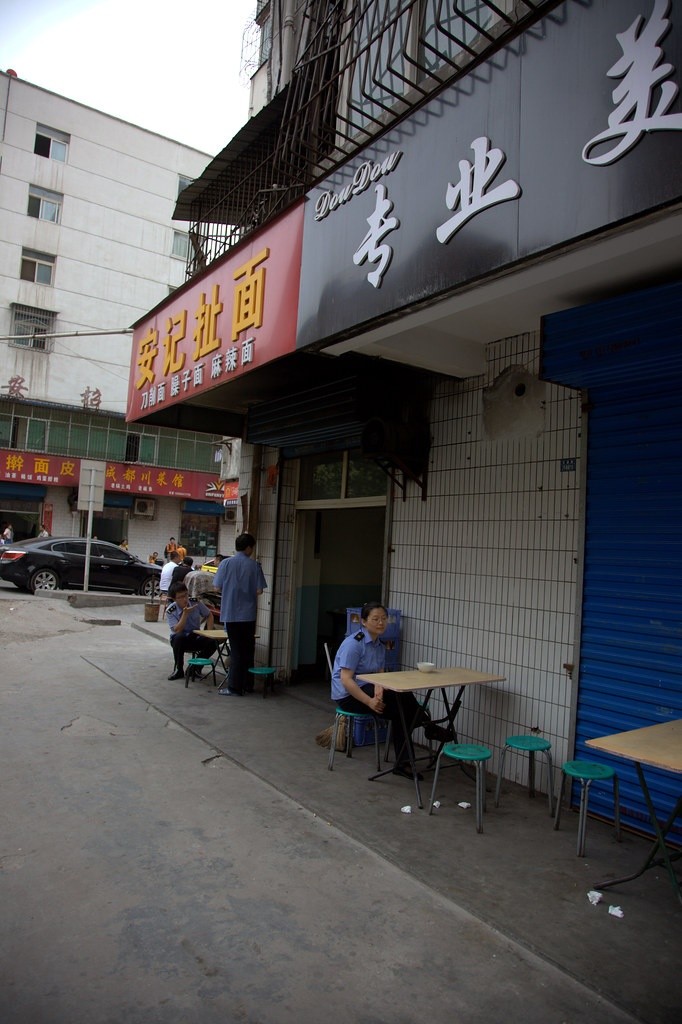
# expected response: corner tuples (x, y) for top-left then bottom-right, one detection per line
(356, 666), (507, 810)
(584, 719), (682, 903)
(193, 629), (260, 690)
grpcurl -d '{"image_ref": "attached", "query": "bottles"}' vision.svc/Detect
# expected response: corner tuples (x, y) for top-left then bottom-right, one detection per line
(353, 615), (397, 650)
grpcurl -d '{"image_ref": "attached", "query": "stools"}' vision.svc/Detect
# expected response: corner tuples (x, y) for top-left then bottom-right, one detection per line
(429, 744), (491, 833)
(495, 735), (555, 816)
(184, 658), (216, 688)
(377, 701), (433, 762)
(553, 761), (622, 857)
(162, 597), (174, 619)
(327, 707), (382, 771)
(248, 667), (279, 699)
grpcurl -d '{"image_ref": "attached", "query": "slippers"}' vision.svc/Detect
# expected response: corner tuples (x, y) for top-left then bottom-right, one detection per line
(218, 688), (241, 696)
(241, 686), (256, 693)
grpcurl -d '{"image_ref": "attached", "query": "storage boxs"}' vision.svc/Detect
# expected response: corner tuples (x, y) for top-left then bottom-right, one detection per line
(343, 607), (401, 748)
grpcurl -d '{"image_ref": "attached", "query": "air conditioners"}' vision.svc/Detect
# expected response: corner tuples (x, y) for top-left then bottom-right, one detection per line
(134, 499), (155, 516)
(224, 509), (237, 521)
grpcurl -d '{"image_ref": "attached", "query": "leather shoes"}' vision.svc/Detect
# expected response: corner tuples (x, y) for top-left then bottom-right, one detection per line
(184, 663), (207, 679)
(168, 670), (184, 680)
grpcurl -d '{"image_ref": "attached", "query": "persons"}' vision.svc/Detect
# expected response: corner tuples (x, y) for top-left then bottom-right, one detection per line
(92, 535), (104, 557)
(149, 537), (223, 606)
(0, 522), (12, 546)
(165, 582), (214, 680)
(212, 534), (268, 696)
(119, 539), (129, 559)
(331, 602), (454, 781)
(38, 525), (48, 537)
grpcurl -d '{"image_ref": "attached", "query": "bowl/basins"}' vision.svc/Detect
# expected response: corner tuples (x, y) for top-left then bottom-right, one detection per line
(417, 661), (434, 672)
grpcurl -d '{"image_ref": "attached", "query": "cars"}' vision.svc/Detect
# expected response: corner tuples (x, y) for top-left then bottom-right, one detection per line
(0, 537), (164, 597)
(201, 554), (234, 573)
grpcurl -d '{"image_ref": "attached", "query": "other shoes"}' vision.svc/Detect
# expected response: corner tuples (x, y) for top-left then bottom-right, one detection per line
(393, 761), (425, 782)
(424, 723), (457, 742)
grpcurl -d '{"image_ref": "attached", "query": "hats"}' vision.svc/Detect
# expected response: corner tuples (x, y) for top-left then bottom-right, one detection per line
(178, 541), (182, 545)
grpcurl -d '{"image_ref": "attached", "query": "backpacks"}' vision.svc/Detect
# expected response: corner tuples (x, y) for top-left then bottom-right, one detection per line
(164, 544), (176, 558)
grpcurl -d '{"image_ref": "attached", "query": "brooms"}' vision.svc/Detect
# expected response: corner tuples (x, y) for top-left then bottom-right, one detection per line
(315, 714), (347, 752)
